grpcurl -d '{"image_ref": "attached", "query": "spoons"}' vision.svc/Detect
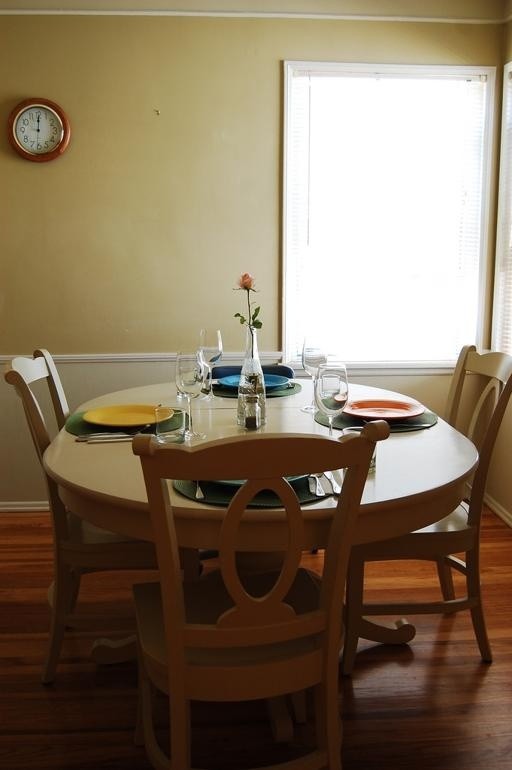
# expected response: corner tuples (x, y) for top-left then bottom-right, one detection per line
(85, 435), (187, 446)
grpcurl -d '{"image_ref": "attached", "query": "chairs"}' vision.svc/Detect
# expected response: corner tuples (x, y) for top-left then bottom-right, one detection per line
(343, 346), (512, 674)
(133, 418), (387, 770)
(5, 347), (198, 686)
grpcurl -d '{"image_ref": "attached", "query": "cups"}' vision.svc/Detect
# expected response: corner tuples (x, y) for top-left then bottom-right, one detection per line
(341, 427), (376, 477)
(154, 404), (184, 445)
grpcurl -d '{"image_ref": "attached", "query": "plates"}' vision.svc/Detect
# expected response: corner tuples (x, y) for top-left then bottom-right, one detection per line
(343, 396), (426, 420)
(82, 405), (177, 427)
(215, 371), (291, 391)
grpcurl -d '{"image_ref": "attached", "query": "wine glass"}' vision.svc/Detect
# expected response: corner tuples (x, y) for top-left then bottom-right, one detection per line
(300, 335), (348, 440)
(174, 327), (225, 444)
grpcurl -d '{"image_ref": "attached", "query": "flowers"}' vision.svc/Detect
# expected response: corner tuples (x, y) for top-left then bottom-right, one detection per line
(232, 273), (263, 363)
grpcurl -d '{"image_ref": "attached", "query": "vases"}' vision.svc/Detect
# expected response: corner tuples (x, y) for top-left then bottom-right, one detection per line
(236, 327), (267, 429)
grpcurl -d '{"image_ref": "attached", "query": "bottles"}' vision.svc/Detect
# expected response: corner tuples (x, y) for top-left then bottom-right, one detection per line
(236, 327), (268, 429)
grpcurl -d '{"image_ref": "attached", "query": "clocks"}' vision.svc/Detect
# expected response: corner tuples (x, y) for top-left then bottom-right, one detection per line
(7, 98), (72, 162)
